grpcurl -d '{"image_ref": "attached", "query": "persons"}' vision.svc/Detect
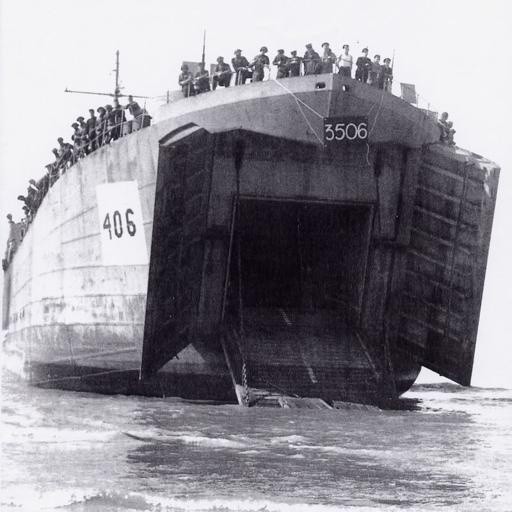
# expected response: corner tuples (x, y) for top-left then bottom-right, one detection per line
(1, 96), (154, 273)
(177, 41), (393, 100)
(444, 120), (455, 135)
(438, 110), (456, 146)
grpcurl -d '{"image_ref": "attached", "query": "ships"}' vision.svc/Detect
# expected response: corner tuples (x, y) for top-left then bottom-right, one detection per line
(0, 29), (501, 410)
